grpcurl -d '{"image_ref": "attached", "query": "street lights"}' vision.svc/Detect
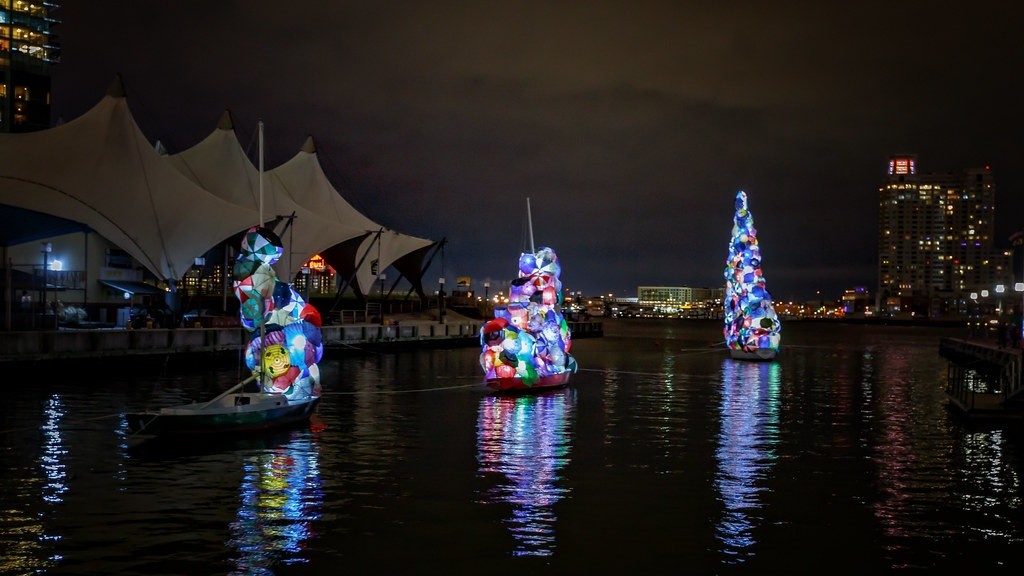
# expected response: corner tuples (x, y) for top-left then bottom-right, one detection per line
(193, 255), (206, 328)
(39, 241), (52, 317)
(969, 292), (979, 338)
(378, 273), (386, 326)
(50, 260), (64, 330)
(438, 275), (445, 324)
(994, 283), (1006, 350)
(483, 281), (490, 323)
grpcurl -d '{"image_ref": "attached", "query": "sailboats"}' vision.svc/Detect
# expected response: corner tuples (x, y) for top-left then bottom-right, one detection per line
(720, 190), (782, 361)
(483, 197), (574, 393)
(123, 120), (322, 439)
